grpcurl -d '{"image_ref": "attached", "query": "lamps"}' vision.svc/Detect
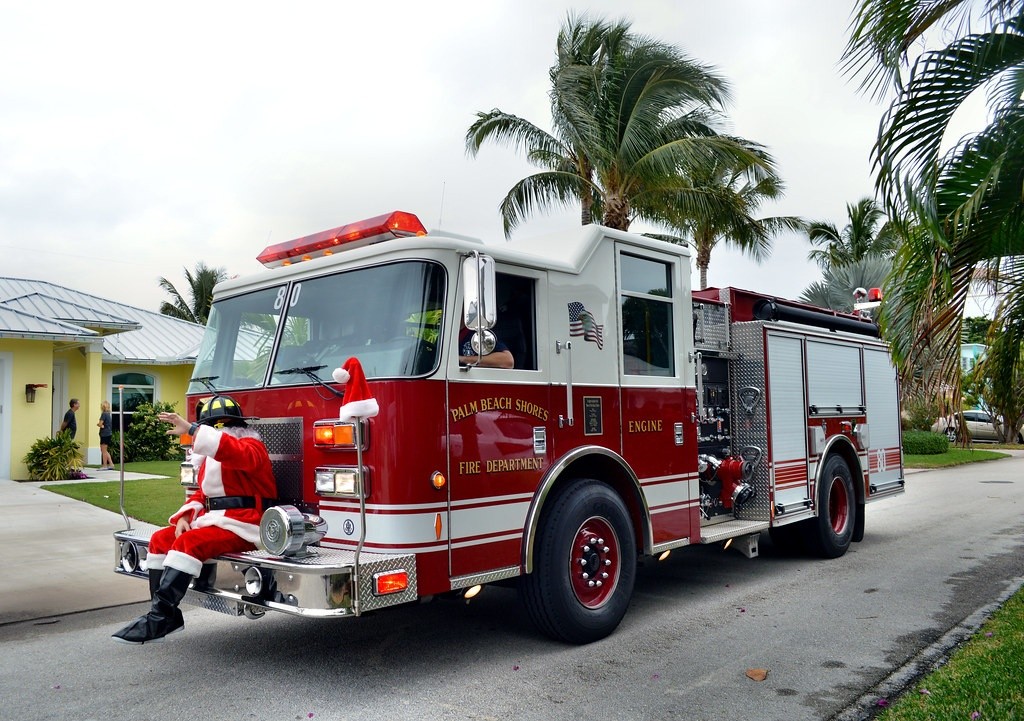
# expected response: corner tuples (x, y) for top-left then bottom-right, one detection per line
(26, 384), (36, 403)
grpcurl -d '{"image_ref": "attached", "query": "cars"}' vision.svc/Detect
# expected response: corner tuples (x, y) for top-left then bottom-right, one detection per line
(930, 409), (1024, 445)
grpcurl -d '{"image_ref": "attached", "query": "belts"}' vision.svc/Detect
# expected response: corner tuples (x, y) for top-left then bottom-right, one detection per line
(205, 496), (277, 513)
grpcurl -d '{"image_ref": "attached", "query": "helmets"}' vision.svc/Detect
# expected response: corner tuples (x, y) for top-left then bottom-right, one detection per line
(196, 395), (260, 425)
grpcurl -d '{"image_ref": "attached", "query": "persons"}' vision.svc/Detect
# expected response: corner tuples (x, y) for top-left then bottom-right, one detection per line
(434, 300), (514, 368)
(61, 399), (81, 440)
(111, 395), (277, 644)
(96, 401), (115, 471)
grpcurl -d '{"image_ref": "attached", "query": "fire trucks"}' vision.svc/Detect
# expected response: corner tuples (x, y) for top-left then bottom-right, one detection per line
(113, 209), (904, 647)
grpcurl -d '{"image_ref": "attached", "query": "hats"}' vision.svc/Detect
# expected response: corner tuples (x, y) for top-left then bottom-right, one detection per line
(332, 356), (379, 422)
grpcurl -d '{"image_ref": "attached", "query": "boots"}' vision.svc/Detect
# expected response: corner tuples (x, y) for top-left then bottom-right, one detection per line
(111, 550), (202, 645)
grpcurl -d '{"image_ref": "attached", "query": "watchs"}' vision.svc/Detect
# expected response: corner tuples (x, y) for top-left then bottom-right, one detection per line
(189, 422), (199, 436)
